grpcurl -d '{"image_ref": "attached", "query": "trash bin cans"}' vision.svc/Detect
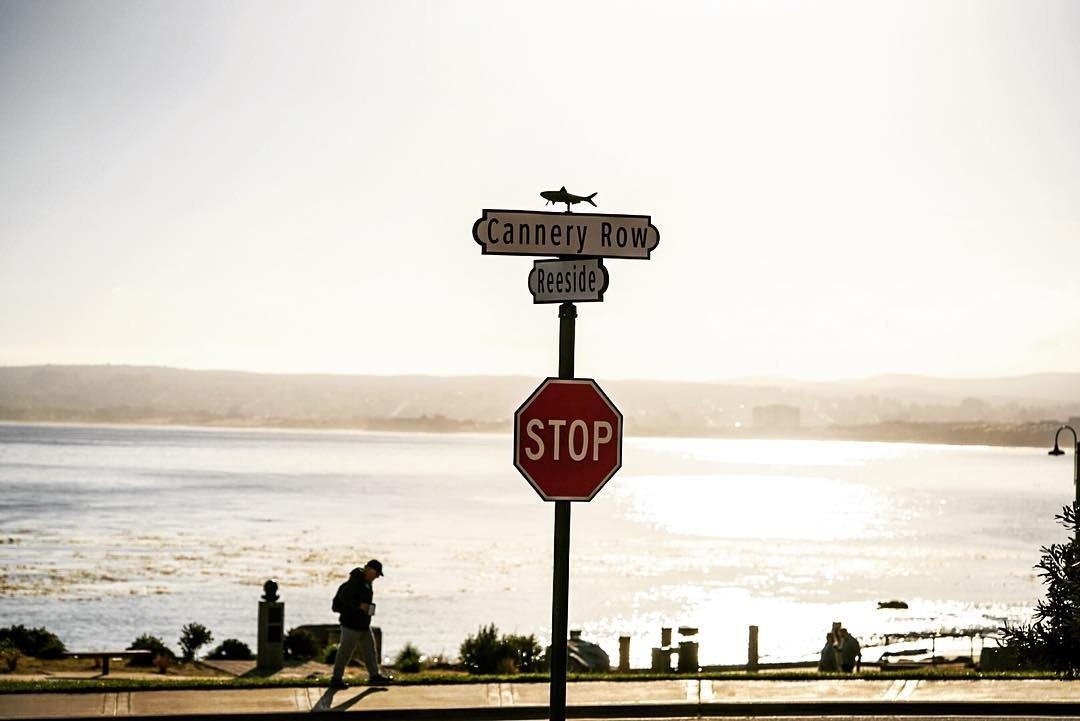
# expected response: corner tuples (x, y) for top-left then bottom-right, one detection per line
(678, 642), (699, 673)
(652, 648), (670, 673)
(258, 580), (285, 671)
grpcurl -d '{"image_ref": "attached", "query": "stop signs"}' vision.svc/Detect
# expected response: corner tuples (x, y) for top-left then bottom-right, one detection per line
(513, 377), (624, 505)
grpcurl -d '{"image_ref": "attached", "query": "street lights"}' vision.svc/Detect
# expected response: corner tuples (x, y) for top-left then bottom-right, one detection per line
(1048, 425), (1080, 543)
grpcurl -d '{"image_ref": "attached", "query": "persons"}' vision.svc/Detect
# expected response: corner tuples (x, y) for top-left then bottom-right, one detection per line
(818, 632), (839, 672)
(328, 560), (395, 688)
(836, 628), (860, 674)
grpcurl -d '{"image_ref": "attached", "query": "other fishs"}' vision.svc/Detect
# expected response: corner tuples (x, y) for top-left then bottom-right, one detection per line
(540, 186), (598, 207)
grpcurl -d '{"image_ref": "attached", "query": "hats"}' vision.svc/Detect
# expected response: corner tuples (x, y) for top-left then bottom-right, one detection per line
(368, 560), (384, 576)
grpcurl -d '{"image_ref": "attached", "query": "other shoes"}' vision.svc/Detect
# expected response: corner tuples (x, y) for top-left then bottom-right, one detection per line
(330, 679), (349, 689)
(371, 674), (394, 682)
